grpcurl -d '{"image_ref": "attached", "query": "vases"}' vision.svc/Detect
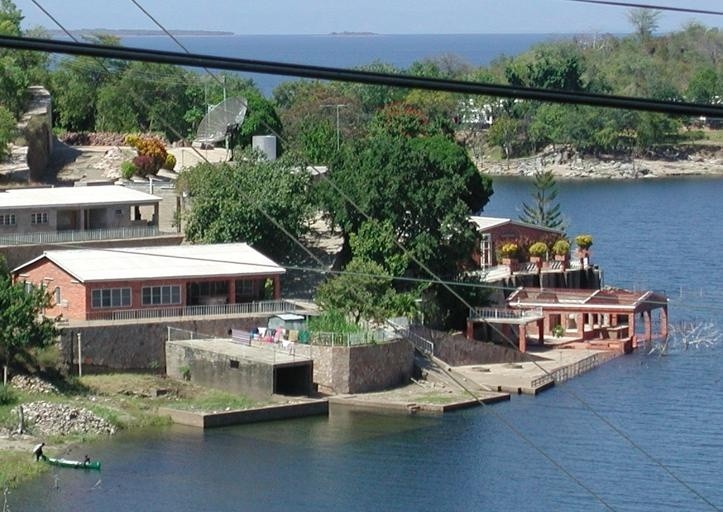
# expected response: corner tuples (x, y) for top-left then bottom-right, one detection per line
(529, 255), (541, 263)
(502, 258), (519, 273)
(555, 253), (572, 261)
(577, 248), (591, 256)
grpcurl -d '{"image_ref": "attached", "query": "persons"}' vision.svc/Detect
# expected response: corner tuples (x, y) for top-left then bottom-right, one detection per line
(31, 442), (47, 461)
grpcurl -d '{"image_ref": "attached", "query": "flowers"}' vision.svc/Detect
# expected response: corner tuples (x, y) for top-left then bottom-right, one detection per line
(551, 239), (570, 254)
(576, 236), (592, 250)
(502, 243), (517, 256)
(529, 240), (549, 256)
